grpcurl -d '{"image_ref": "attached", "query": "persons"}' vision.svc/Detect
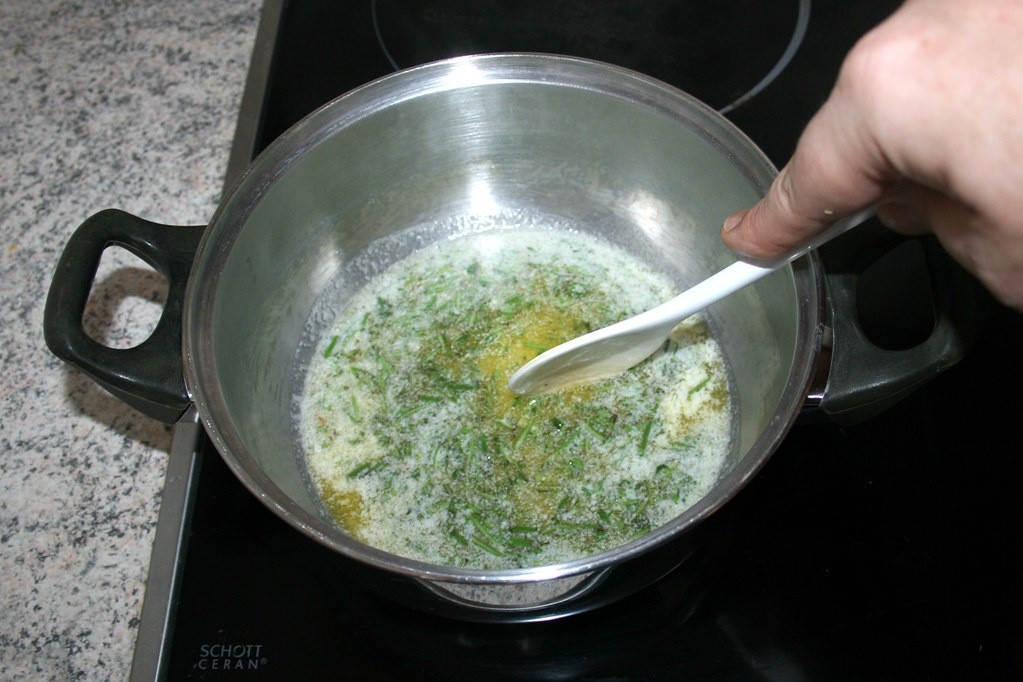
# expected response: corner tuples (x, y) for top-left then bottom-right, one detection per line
(721, 0), (1023, 312)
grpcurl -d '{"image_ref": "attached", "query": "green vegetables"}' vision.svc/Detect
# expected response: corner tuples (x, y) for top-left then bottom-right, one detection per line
(321, 243), (717, 565)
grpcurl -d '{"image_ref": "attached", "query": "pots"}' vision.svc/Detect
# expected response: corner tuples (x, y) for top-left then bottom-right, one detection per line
(42, 49), (980, 622)
